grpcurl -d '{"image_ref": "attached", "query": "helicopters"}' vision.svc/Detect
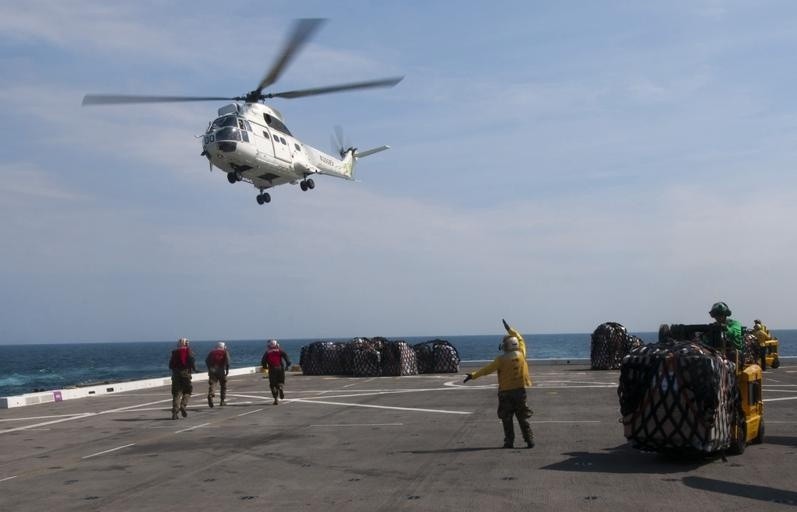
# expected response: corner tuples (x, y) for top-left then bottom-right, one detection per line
(77, 15), (406, 203)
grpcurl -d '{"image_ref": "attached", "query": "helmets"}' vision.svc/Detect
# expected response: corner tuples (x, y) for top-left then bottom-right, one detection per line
(214, 342), (225, 350)
(504, 336), (519, 351)
(178, 338), (189, 347)
(267, 339), (276, 348)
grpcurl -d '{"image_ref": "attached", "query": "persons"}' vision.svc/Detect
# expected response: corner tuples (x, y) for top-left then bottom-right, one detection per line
(260, 339), (290, 406)
(751, 320), (768, 335)
(169, 338), (202, 419)
(700, 301), (745, 369)
(204, 342), (229, 407)
(463, 319), (535, 448)
(750, 325), (772, 368)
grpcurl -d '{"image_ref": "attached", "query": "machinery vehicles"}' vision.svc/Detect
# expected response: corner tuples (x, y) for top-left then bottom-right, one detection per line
(617, 322), (772, 460)
(736, 316), (785, 369)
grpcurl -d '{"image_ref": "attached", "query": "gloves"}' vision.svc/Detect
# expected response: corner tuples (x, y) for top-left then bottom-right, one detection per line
(502, 318), (509, 330)
(463, 373), (471, 383)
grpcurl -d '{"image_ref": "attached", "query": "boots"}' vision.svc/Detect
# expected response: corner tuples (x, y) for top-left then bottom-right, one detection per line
(171, 405), (178, 420)
(208, 394), (214, 407)
(220, 398), (225, 406)
(179, 403), (187, 418)
(279, 386), (284, 399)
(272, 397), (278, 405)
